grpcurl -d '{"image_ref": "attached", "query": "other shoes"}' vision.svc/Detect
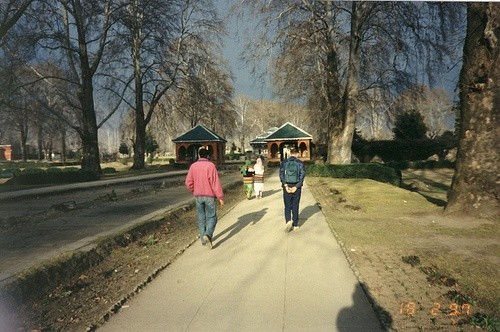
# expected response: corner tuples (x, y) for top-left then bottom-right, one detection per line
(203, 234), (213, 249)
(202, 242), (206, 246)
(284, 221), (292, 233)
(293, 226), (298, 230)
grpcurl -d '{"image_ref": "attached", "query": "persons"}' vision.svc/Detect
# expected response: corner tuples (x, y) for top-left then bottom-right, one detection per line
(278, 148), (307, 233)
(248, 157), (266, 199)
(184, 148), (225, 249)
(241, 159), (255, 200)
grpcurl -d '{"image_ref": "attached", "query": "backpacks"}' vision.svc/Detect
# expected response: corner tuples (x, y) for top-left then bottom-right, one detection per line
(283, 158), (299, 183)
